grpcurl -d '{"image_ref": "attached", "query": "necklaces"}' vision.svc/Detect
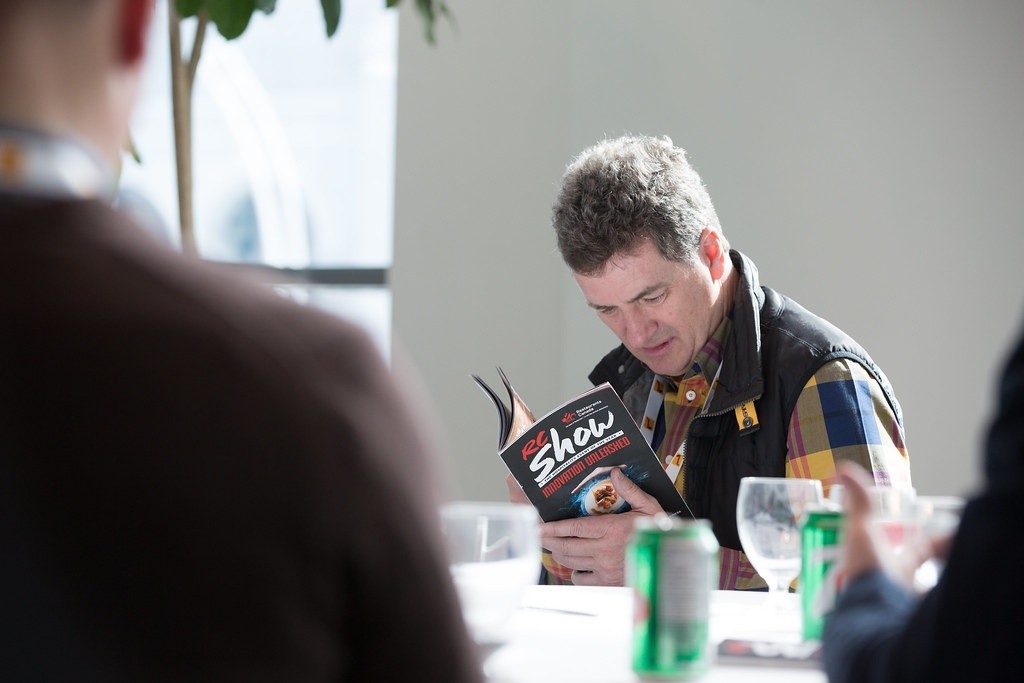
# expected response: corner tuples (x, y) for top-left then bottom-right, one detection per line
(0, 121), (105, 199)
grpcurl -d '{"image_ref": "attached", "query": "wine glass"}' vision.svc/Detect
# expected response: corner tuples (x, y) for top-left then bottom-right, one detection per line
(736, 477), (823, 620)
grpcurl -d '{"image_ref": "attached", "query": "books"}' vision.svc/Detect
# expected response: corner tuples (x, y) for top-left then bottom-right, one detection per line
(472, 364), (695, 522)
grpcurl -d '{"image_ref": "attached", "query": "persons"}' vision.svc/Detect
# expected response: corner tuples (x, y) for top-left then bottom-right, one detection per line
(0, 0), (499, 683)
(536, 131), (911, 592)
(825, 332), (1024, 683)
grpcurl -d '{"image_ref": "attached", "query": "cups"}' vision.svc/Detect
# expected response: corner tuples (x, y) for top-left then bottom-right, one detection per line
(828, 483), (968, 593)
(435, 498), (546, 636)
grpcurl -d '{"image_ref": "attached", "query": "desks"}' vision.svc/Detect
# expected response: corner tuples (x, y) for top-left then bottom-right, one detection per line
(454, 572), (829, 683)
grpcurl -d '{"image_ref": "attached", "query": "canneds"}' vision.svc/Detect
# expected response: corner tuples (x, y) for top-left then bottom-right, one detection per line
(626, 514), (719, 683)
(800, 507), (844, 642)
